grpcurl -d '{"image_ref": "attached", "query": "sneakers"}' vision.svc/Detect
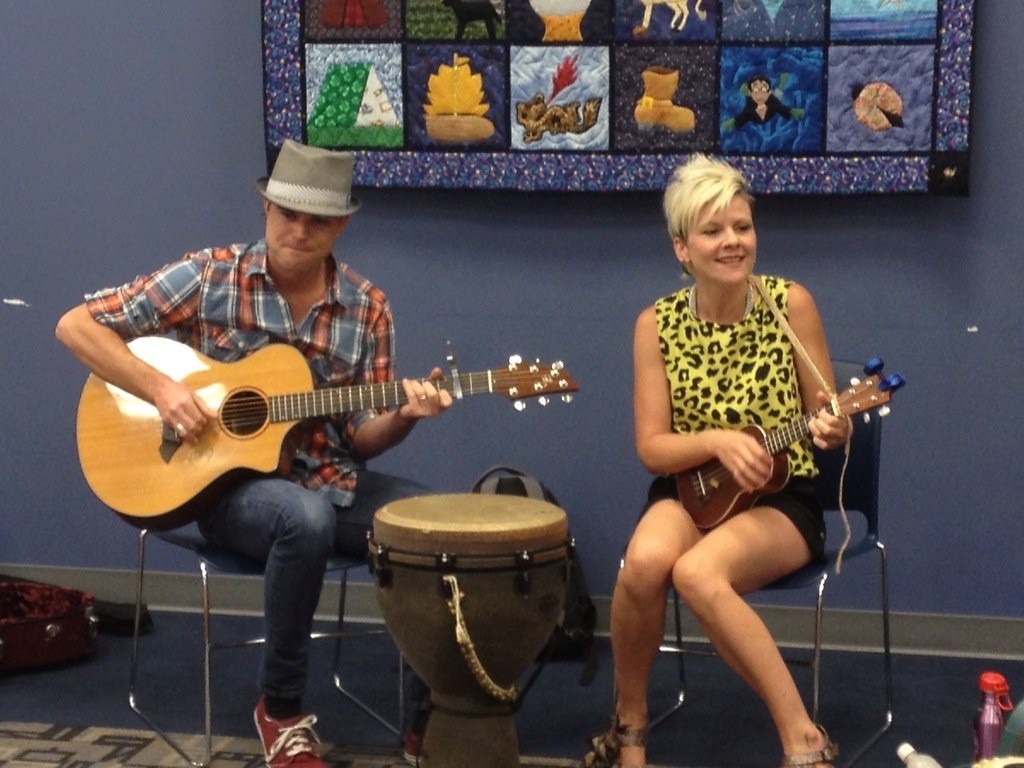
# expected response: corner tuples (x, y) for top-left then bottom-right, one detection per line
(254, 693), (327, 768)
(403, 729), (424, 766)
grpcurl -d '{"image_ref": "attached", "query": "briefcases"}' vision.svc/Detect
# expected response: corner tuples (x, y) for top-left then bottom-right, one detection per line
(0, 575), (101, 673)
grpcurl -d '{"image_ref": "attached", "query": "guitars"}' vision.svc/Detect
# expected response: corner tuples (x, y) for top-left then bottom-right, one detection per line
(675, 355), (906, 531)
(73, 331), (580, 535)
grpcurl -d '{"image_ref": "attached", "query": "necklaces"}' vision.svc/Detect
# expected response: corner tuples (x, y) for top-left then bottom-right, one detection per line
(689, 283), (753, 318)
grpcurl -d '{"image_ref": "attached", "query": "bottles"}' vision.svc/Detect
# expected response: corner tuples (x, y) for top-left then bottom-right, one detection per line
(973, 672), (1013, 762)
(896, 742), (943, 768)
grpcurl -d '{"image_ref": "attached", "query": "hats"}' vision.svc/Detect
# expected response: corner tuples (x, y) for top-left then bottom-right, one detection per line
(256, 139), (362, 216)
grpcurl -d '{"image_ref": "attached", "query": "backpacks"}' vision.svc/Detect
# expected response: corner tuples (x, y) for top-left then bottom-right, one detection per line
(471, 463), (595, 656)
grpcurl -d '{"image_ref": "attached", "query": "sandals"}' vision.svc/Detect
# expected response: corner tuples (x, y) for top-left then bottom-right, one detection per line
(610, 714), (648, 768)
(782, 723), (839, 768)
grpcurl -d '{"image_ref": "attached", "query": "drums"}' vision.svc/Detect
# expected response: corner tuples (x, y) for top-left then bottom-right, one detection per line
(365, 492), (571, 768)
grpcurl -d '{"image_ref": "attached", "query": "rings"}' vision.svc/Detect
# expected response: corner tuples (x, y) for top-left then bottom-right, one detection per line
(175, 424), (183, 431)
(420, 395), (426, 401)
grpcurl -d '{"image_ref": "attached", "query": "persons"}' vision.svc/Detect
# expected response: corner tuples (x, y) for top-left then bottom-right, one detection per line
(53, 140), (452, 768)
(581, 152), (853, 768)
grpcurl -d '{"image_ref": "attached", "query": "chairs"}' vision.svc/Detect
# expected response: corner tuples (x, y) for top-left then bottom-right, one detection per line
(646, 361), (892, 768)
(128, 523), (408, 768)
